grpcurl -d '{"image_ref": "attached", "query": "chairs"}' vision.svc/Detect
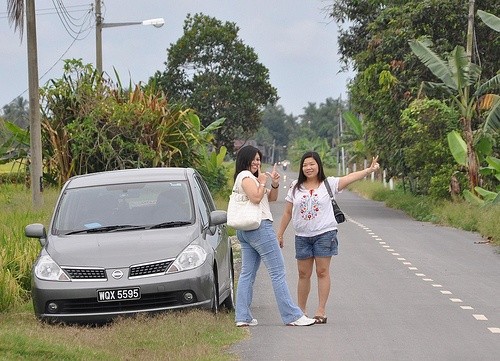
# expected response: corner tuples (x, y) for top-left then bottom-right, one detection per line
(91, 195), (129, 213)
(157, 189), (185, 209)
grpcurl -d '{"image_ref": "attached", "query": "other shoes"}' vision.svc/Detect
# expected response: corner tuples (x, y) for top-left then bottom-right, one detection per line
(288, 314), (316, 326)
(236, 318), (258, 326)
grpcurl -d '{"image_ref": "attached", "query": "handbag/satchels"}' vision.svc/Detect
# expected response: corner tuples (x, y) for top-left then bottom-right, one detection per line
(324, 178), (345, 223)
(226, 172), (261, 231)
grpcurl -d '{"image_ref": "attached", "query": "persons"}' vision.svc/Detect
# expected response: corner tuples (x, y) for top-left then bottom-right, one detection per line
(277, 151), (381, 325)
(227, 144), (316, 327)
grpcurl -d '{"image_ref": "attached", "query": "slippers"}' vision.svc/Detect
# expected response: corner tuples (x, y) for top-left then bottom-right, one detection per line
(313, 316), (327, 324)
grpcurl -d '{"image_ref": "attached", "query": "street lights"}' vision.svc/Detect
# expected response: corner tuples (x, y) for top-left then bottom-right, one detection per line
(96, 19), (166, 96)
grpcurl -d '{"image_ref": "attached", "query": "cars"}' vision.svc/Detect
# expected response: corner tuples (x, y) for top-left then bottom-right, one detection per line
(23, 168), (236, 327)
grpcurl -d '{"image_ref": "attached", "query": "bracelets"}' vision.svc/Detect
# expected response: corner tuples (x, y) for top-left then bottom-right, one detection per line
(271, 184), (279, 189)
(259, 184), (266, 187)
